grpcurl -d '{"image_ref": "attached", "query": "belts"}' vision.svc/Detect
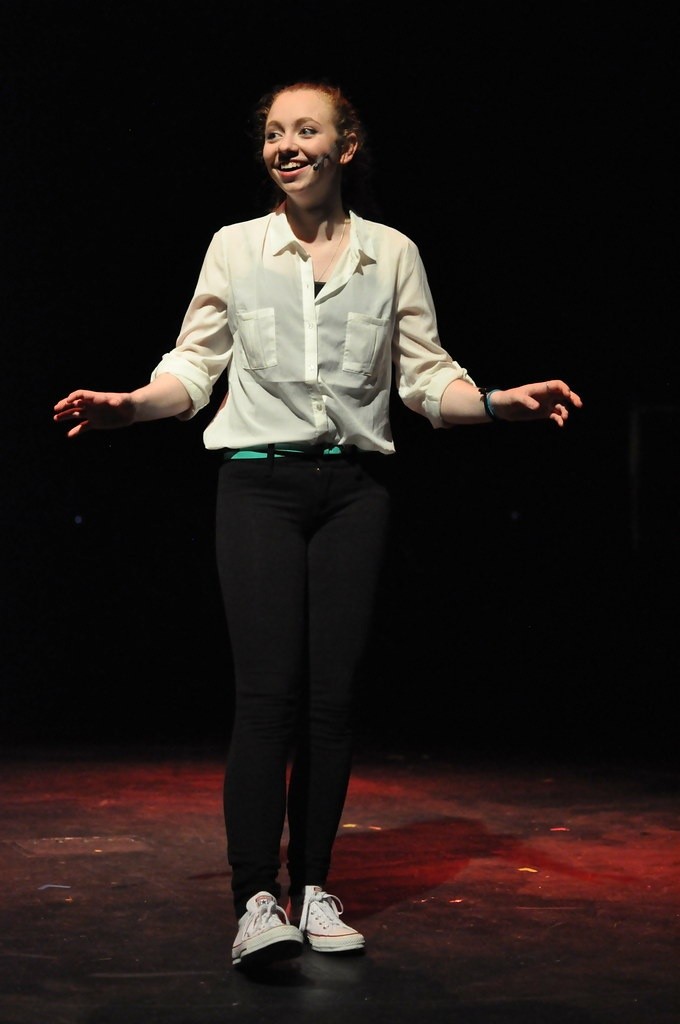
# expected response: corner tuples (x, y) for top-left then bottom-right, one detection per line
(222, 444), (351, 460)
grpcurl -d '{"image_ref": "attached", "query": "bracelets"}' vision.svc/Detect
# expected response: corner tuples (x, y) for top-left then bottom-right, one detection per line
(479, 386), (503, 422)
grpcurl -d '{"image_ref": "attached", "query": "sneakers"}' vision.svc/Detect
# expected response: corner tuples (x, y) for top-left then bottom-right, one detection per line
(286, 885), (365, 952)
(232, 891), (305, 965)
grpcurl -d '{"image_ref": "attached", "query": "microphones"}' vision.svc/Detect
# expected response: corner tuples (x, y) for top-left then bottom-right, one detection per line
(312, 133), (350, 170)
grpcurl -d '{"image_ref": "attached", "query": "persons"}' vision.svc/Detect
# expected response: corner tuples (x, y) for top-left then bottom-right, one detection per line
(54, 83), (581, 968)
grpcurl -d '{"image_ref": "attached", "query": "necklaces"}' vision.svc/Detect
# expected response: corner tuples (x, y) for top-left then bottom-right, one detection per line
(317, 215), (347, 281)
